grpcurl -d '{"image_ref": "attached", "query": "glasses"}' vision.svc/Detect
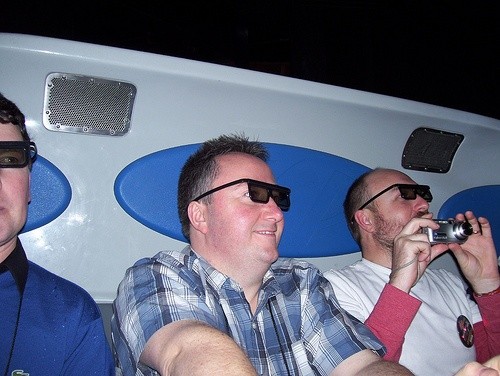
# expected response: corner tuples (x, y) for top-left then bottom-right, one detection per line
(0, 141), (37, 168)
(193, 179), (291, 212)
(350, 184), (433, 225)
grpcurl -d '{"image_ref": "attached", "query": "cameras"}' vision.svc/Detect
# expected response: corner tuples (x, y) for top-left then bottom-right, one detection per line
(420, 218), (473, 245)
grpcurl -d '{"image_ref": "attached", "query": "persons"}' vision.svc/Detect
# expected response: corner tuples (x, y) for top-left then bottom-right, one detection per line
(0, 93), (116, 376)
(111, 134), (415, 376)
(323, 167), (500, 376)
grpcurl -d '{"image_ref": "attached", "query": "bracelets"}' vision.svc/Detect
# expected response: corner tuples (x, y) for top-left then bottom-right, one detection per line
(475, 286), (500, 297)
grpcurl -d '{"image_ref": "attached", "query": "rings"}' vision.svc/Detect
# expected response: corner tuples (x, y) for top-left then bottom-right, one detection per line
(472, 230), (481, 234)
